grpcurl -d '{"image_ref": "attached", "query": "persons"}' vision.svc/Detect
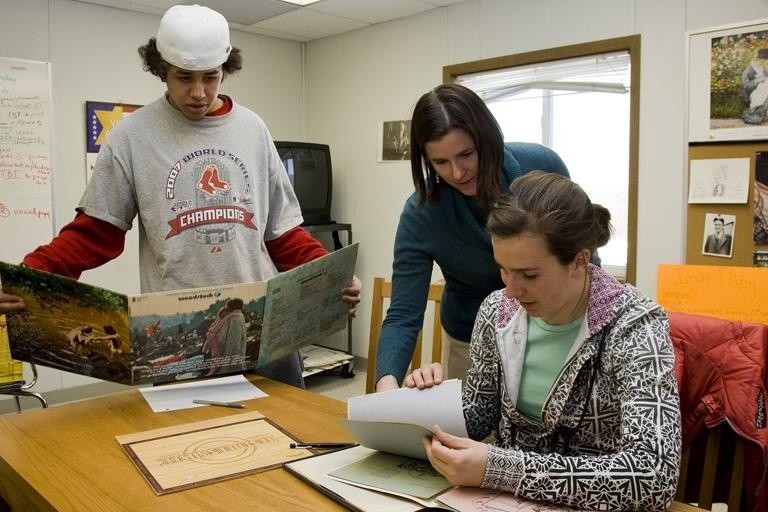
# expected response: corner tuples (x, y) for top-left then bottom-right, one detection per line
(704, 216), (731, 255)
(0, 4), (362, 389)
(94, 325), (123, 361)
(217, 298), (247, 372)
(383, 121), (410, 160)
(742, 48), (768, 108)
(70, 326), (93, 353)
(374, 84), (601, 441)
(403, 170), (684, 512)
(206, 308), (241, 375)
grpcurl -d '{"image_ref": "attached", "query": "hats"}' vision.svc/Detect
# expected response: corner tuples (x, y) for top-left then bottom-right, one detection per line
(155, 5), (232, 72)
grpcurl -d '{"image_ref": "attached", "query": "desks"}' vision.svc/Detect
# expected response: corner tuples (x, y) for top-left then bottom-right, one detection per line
(0, 372), (716, 512)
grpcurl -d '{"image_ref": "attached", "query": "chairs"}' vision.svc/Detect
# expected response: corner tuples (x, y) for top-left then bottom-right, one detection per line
(0, 313), (48, 411)
(365, 276), (446, 396)
(664, 309), (768, 511)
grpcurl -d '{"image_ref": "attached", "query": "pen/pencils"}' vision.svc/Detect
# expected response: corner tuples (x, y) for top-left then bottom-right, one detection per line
(193, 400), (246, 408)
(290, 442), (355, 448)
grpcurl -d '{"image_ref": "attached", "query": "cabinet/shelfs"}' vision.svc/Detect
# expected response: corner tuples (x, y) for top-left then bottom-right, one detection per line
(300, 222), (356, 379)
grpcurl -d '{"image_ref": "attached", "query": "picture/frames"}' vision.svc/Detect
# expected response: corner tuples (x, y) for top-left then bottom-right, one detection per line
(685, 17), (768, 141)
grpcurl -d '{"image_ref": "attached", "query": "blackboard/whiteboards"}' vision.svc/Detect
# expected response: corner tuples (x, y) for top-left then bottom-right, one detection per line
(0, 57), (54, 265)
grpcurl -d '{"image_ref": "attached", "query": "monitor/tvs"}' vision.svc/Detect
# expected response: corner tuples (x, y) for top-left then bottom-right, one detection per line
(274, 141), (332, 226)
